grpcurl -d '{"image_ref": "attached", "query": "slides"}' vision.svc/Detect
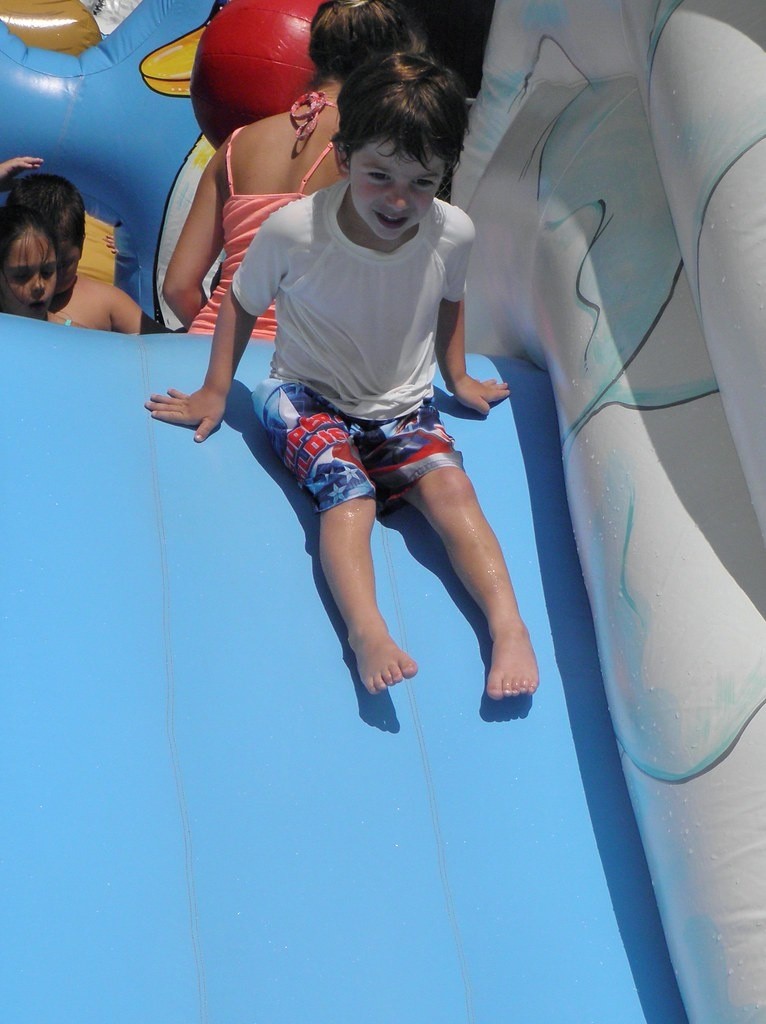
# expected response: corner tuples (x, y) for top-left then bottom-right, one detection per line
(0, 312), (766, 1024)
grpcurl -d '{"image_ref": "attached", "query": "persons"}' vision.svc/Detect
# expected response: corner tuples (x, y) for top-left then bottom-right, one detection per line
(163, 0), (427, 341)
(146, 54), (538, 700)
(0, 204), (86, 328)
(0, 157), (174, 334)
